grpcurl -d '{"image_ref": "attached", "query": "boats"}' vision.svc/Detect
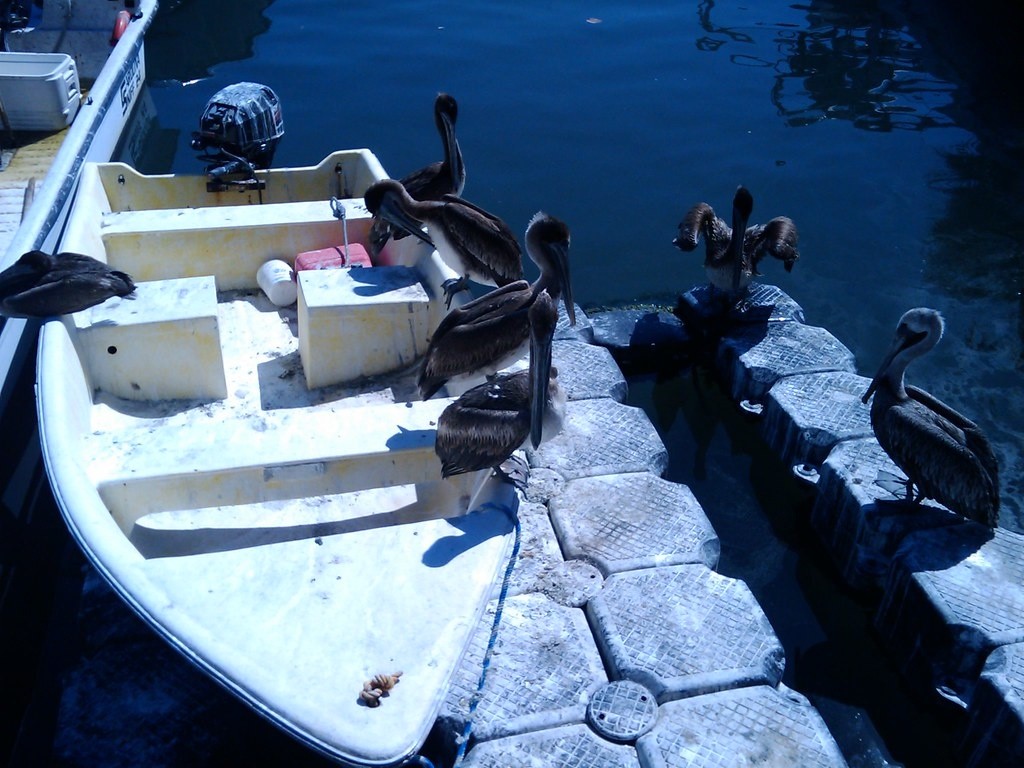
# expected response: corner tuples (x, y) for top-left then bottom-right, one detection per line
(34, 82), (537, 767)
(1, 0), (159, 487)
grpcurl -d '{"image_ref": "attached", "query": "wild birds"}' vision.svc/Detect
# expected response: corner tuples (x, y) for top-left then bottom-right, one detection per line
(434, 289), (558, 501)
(415, 209), (576, 401)
(671, 184), (799, 306)
(861, 307), (1000, 528)
(0, 250), (137, 325)
(364, 179), (525, 310)
(368, 94), (466, 255)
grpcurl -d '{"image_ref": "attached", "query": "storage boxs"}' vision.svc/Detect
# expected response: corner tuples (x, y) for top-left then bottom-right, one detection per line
(0, 52), (81, 131)
